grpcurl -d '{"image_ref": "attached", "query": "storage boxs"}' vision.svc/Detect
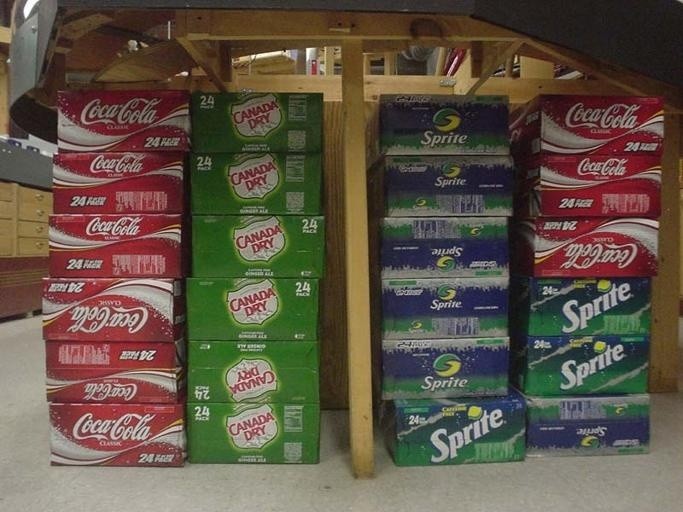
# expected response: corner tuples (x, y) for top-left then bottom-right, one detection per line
(370, 278), (511, 340)
(512, 387), (650, 458)
(44, 340), (187, 404)
(186, 403), (321, 466)
(190, 214), (326, 280)
(370, 218), (512, 278)
(512, 217), (660, 278)
(53, 152), (189, 213)
(370, 340), (511, 400)
(55, 89), (193, 154)
(190, 92), (325, 155)
(48, 403), (187, 469)
(39, 278), (186, 344)
(511, 93), (667, 163)
(367, 156), (514, 217)
(510, 275), (652, 337)
(188, 340), (320, 405)
(511, 154), (665, 221)
(365, 92), (511, 174)
(185, 278), (320, 340)
(48, 214), (191, 280)
(377, 385), (527, 467)
(187, 153), (324, 215)
(511, 331), (649, 397)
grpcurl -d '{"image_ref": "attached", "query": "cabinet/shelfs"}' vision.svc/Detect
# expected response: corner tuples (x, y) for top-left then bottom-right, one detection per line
(8, 0), (681, 481)
(16, 186), (53, 258)
(0, 180), (15, 259)
